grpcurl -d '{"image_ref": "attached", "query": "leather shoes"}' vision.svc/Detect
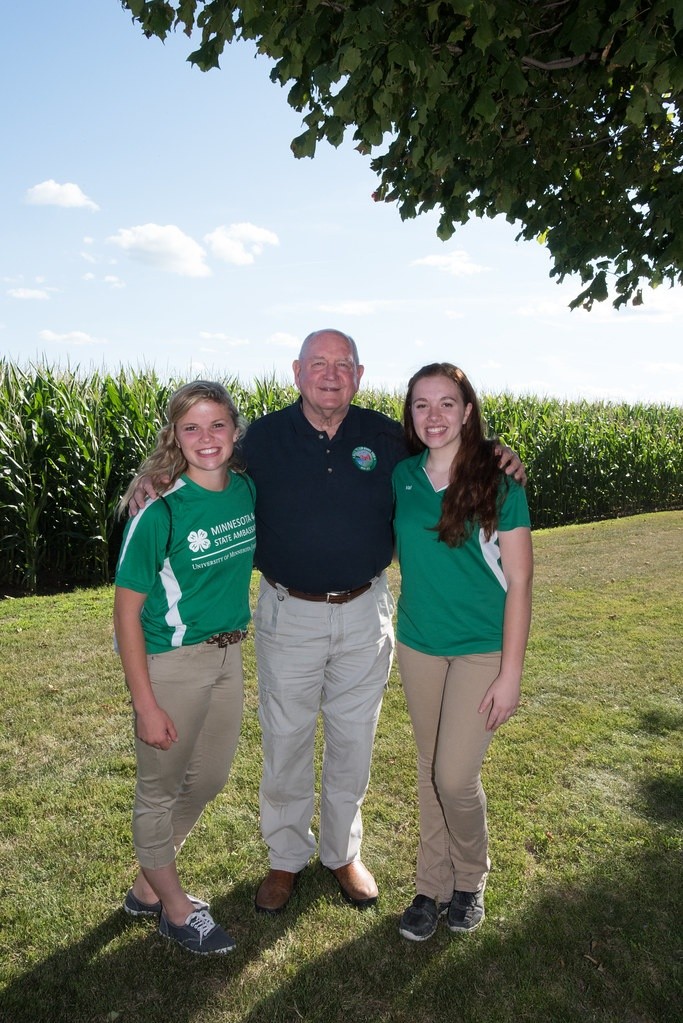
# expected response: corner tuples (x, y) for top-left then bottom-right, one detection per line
(256, 870), (297, 912)
(334, 860), (380, 902)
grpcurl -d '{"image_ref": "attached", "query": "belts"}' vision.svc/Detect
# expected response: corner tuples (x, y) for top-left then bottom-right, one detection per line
(264, 574), (381, 604)
(206, 630), (249, 648)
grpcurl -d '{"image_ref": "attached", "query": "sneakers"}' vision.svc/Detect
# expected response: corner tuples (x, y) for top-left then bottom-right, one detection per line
(449, 889), (489, 934)
(123, 886), (210, 918)
(159, 908), (236, 955)
(399, 894), (451, 942)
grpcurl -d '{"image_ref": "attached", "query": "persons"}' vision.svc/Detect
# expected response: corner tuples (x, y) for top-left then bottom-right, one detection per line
(391, 363), (534, 941)
(126, 328), (528, 911)
(113, 380), (257, 955)
